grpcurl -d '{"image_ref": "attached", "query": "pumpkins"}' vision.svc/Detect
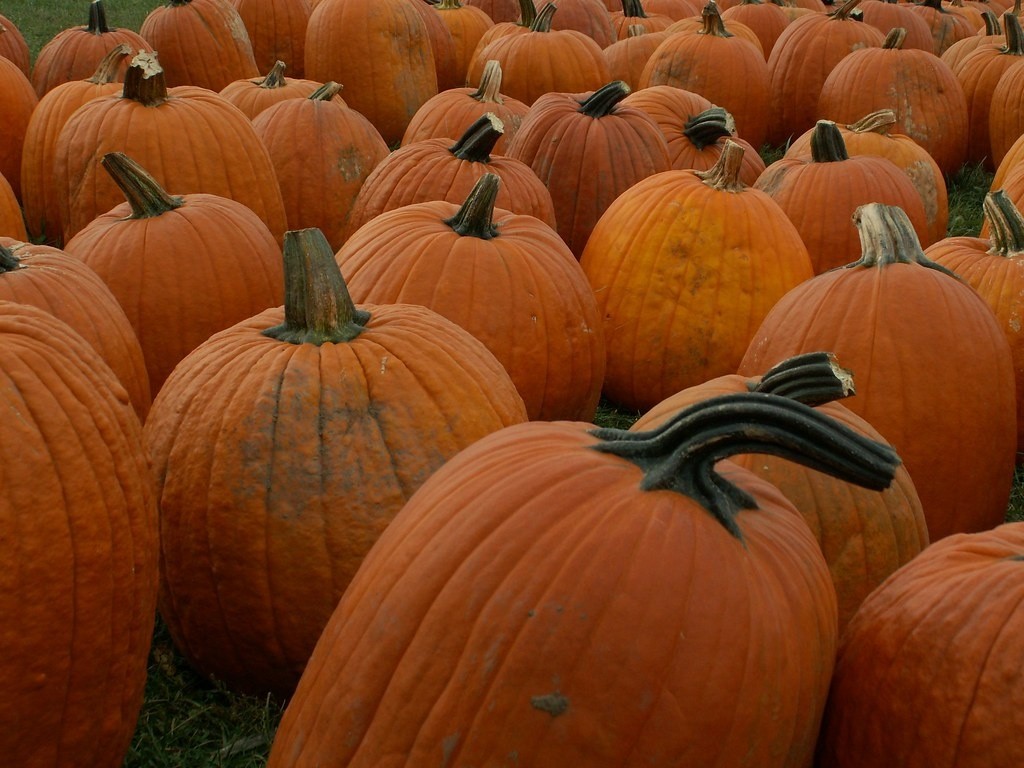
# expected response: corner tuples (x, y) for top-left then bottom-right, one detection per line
(0, 0), (1024, 768)
